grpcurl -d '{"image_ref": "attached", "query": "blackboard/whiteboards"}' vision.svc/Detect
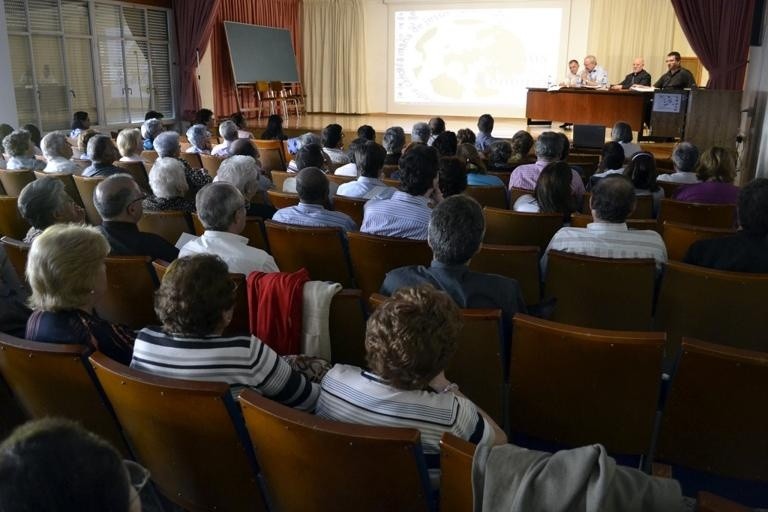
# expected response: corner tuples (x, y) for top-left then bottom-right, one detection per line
(223, 21), (301, 84)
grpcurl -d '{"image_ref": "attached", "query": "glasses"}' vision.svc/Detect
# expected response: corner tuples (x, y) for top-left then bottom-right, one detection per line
(123, 458), (151, 505)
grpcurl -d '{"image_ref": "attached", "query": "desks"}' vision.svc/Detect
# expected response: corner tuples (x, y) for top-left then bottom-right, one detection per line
(522, 85), (664, 142)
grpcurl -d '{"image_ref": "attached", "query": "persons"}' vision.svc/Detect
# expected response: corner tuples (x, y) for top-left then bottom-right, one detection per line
(569, 55), (608, 87)
(559, 60), (579, 128)
(606, 57), (651, 90)
(633, 51), (698, 90)
(0, 103), (768, 512)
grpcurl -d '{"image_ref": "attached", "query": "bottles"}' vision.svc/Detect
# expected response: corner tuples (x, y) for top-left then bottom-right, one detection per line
(547, 75), (551, 87)
(575, 72), (582, 87)
(601, 73), (607, 89)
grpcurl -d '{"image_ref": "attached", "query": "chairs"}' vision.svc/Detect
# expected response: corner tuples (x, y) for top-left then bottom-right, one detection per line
(659, 334), (768, 509)
(0, 336), (165, 511)
(270, 82), (306, 118)
(86, 353), (267, 510)
(434, 432), (691, 510)
(253, 79), (285, 119)
(1, 132), (768, 423)
(236, 390), (429, 510)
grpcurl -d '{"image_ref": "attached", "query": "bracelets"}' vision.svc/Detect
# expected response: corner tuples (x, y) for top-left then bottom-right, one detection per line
(586, 79), (588, 85)
